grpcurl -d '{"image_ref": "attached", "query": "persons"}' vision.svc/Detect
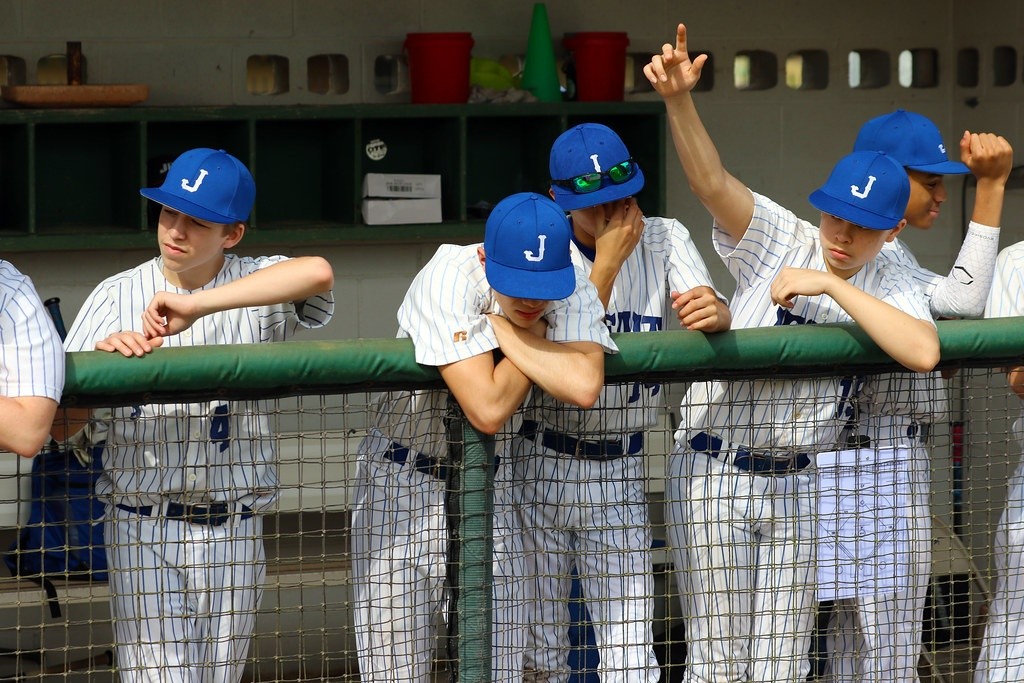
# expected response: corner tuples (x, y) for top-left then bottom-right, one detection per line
(49, 147), (334, 683)
(351, 122), (732, 683)
(644, 23), (1024, 683)
(0, 258), (66, 458)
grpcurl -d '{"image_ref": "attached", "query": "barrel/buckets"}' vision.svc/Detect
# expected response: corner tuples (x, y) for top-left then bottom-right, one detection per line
(403, 32), (475, 103)
(561, 31), (630, 100)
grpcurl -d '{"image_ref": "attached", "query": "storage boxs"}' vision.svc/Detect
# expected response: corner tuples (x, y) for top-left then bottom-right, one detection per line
(4, 83), (150, 110)
(363, 172), (443, 200)
(361, 198), (443, 226)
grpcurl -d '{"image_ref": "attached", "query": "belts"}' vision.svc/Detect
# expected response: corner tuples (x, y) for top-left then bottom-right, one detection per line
(115, 500), (252, 526)
(839, 411), (931, 442)
(382, 441), (500, 482)
(517, 418), (642, 461)
(689, 432), (810, 476)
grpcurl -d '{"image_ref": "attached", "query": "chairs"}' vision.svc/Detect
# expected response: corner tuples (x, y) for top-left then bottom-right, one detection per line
(920, 513), (992, 683)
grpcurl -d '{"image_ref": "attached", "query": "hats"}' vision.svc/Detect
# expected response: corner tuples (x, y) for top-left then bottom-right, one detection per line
(853, 108), (971, 174)
(807, 150), (908, 231)
(549, 124), (645, 212)
(485, 192), (576, 301)
(140, 149), (255, 223)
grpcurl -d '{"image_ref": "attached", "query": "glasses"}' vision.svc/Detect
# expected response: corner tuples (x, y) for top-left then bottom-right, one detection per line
(549, 157), (635, 194)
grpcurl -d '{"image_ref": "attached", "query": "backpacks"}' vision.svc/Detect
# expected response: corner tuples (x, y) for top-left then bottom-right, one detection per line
(3, 441), (110, 580)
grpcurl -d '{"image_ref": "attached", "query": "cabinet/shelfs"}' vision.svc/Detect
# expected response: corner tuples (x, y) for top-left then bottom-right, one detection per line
(0, 101), (669, 251)
